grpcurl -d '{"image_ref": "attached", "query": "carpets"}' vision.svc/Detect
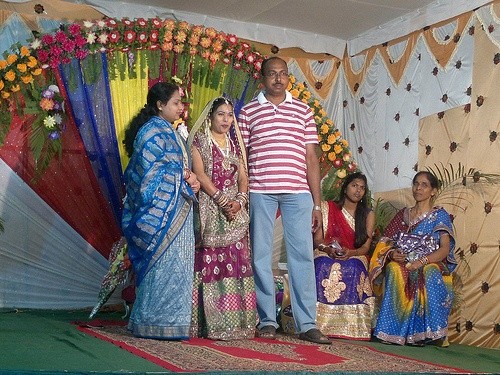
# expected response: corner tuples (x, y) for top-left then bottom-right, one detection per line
(72, 321), (479, 374)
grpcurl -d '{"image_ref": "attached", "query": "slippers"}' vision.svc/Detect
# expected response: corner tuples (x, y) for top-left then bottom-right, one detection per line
(258, 325), (276, 340)
(299, 328), (332, 344)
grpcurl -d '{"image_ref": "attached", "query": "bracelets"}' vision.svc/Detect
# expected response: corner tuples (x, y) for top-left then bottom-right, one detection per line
(318, 243), (327, 252)
(184, 168), (191, 181)
(235, 192), (250, 208)
(419, 256), (430, 266)
(313, 205), (322, 212)
(212, 191), (229, 209)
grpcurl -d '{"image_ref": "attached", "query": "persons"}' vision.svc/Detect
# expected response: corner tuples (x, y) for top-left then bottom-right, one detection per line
(189, 96), (257, 339)
(364, 172), (457, 345)
(241, 57), (333, 344)
(312, 172), (376, 340)
(121, 83), (201, 337)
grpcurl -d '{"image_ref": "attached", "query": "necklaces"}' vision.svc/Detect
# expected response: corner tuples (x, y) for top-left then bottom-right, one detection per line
(210, 131), (231, 170)
(409, 209), (433, 226)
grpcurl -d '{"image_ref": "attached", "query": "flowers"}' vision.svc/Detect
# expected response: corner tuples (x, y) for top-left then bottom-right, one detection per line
(0, 17), (372, 202)
